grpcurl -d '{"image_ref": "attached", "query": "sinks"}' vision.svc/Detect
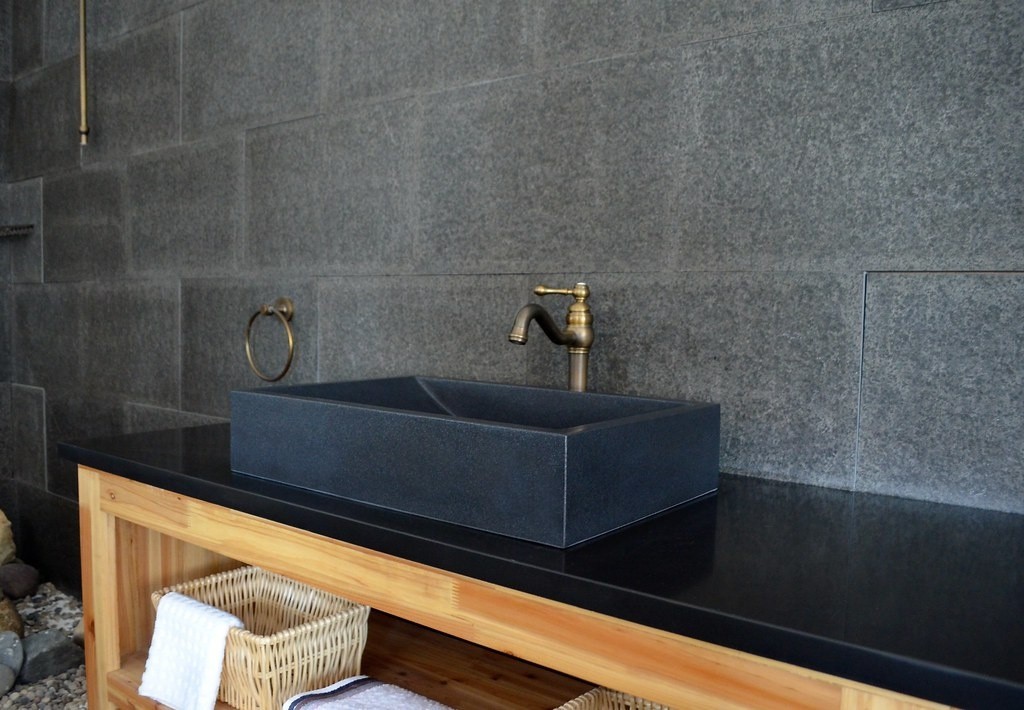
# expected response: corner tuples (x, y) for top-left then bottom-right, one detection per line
(227, 373), (723, 549)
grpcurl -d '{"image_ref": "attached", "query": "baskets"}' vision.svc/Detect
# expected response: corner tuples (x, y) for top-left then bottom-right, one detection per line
(548, 686), (676, 710)
(149, 565), (373, 709)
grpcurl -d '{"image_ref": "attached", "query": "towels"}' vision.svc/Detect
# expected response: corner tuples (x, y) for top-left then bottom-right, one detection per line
(280, 675), (457, 710)
(137, 591), (246, 710)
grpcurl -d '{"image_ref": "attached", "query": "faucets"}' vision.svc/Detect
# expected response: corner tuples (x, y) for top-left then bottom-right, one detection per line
(506, 281), (595, 395)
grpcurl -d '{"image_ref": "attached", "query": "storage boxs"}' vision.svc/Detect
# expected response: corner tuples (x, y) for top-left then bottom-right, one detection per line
(552, 686), (671, 710)
(150, 562), (371, 710)
